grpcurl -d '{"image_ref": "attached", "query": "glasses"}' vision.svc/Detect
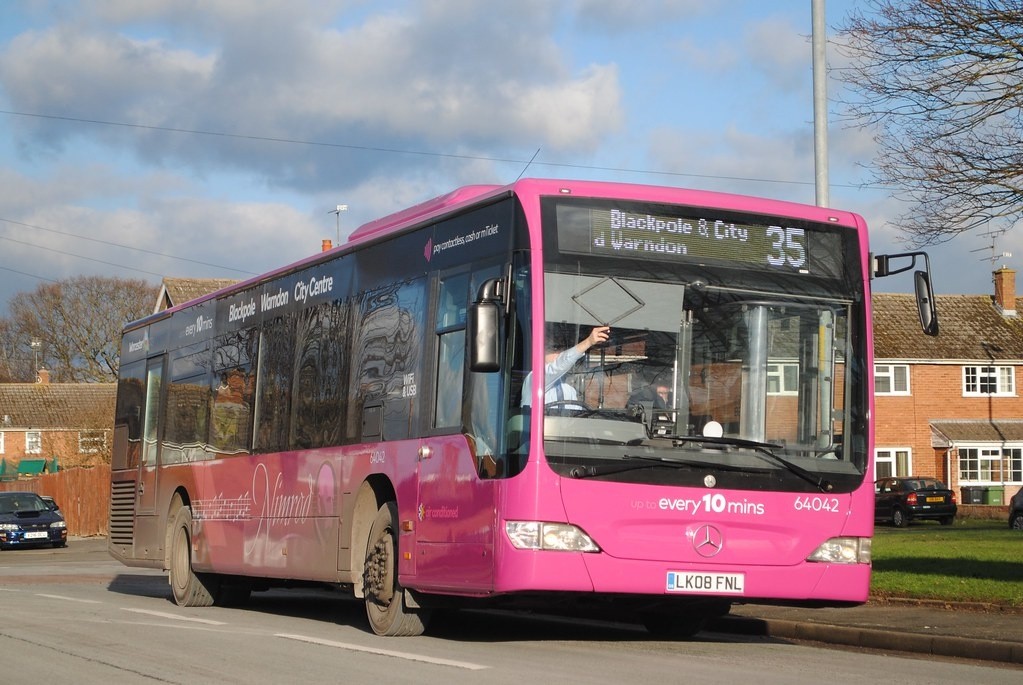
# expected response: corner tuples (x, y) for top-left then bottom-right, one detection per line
(546, 344), (566, 351)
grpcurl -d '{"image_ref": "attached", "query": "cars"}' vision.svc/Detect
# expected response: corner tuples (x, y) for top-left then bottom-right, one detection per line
(1008, 485), (1023, 530)
(875, 476), (958, 528)
(0, 491), (68, 550)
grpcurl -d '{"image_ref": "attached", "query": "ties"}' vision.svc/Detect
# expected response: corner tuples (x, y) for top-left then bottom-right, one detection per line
(555, 379), (565, 409)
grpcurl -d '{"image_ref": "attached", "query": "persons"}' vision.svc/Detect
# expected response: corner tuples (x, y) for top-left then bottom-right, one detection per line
(626, 367), (674, 435)
(520, 326), (611, 411)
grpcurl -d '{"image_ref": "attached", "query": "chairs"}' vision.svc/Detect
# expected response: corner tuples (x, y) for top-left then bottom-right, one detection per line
(927, 485), (935, 490)
(890, 485), (898, 492)
(18, 502), (33, 510)
(910, 483), (918, 490)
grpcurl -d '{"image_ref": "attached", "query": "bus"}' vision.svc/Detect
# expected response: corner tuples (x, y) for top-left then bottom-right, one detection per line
(108, 177), (940, 637)
